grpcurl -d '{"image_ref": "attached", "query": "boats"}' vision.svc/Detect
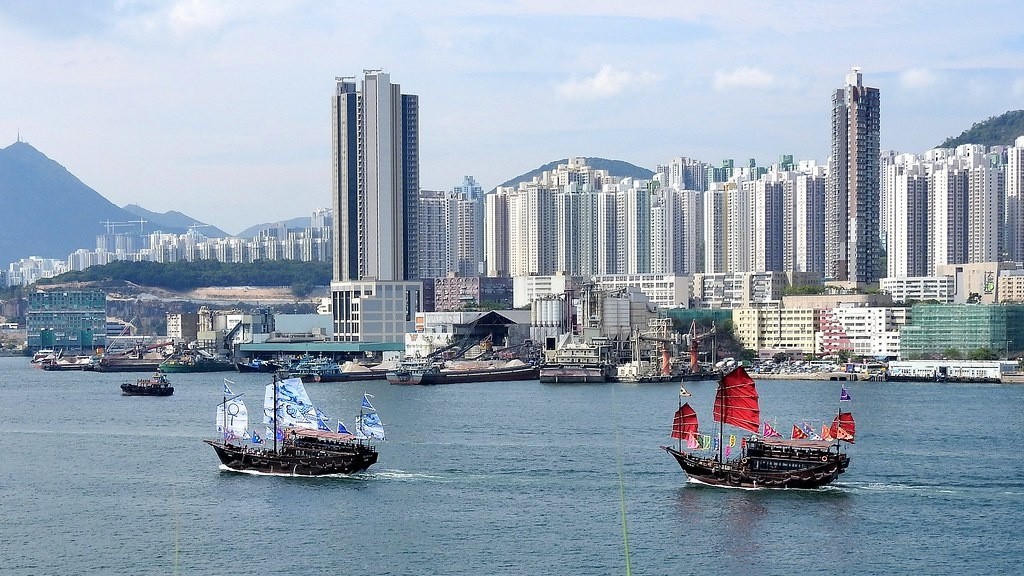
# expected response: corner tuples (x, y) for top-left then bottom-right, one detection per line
(204, 369), (388, 480)
(31, 348), (159, 371)
(158, 346), (387, 382)
(661, 365), (855, 491)
(386, 346), (539, 385)
(120, 372), (175, 397)
(538, 335), (622, 382)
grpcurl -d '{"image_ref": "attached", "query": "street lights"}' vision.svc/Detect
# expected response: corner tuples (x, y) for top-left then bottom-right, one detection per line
(1006, 340), (1013, 361)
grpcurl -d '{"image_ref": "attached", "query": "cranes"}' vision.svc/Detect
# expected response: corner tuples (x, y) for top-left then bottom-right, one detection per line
(632, 319), (717, 376)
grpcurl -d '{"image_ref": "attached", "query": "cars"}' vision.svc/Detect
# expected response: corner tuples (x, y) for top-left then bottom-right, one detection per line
(720, 358), (885, 375)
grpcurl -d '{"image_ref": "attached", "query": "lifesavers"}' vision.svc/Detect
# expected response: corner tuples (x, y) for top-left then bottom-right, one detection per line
(742, 458), (747, 463)
(822, 456), (827, 462)
